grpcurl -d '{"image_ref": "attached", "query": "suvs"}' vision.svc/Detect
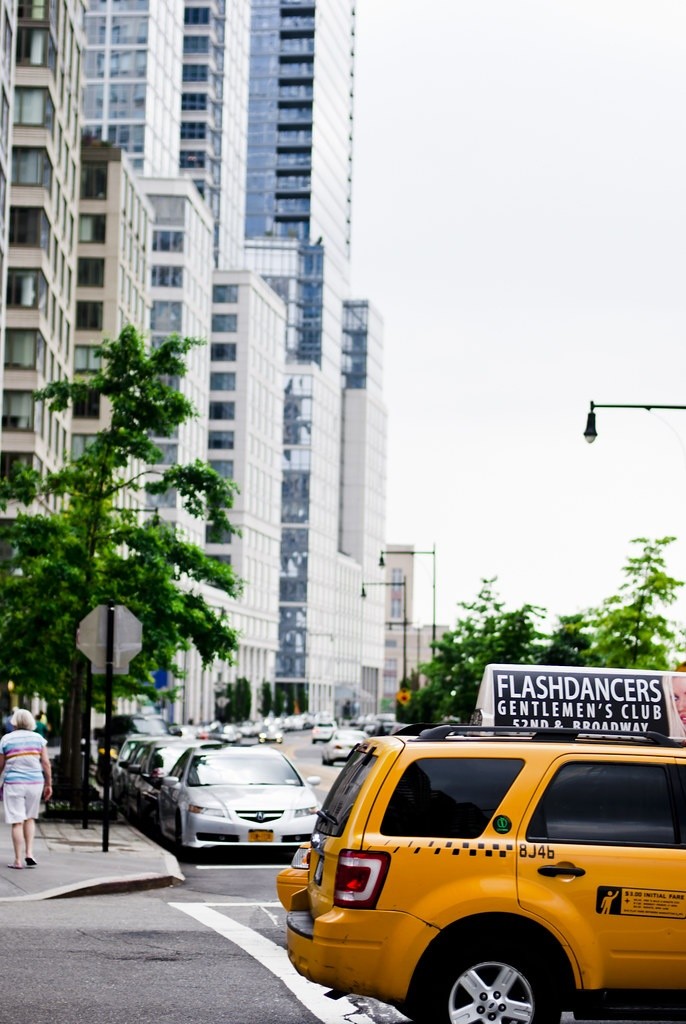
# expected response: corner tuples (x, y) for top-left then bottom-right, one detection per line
(276, 723), (686, 1024)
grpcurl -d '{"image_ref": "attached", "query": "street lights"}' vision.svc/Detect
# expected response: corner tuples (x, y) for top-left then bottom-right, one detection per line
(378, 541), (436, 658)
(360, 575), (407, 681)
(584, 400), (686, 444)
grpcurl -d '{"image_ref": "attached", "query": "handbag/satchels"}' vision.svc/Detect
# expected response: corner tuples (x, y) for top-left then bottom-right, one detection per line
(0, 783), (4, 802)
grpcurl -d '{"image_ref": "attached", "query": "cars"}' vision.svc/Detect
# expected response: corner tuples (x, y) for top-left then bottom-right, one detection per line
(194, 710), (338, 746)
(127, 739), (225, 836)
(159, 748), (323, 863)
(277, 842), (313, 911)
(93, 715), (183, 808)
(321, 731), (371, 766)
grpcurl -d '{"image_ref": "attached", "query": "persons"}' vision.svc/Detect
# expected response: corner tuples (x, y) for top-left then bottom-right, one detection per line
(2, 706), (51, 738)
(0, 709), (52, 869)
(662, 676), (686, 742)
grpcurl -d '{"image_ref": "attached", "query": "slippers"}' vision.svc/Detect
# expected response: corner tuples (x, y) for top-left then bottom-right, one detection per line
(7, 861), (24, 872)
(25, 856), (37, 868)
